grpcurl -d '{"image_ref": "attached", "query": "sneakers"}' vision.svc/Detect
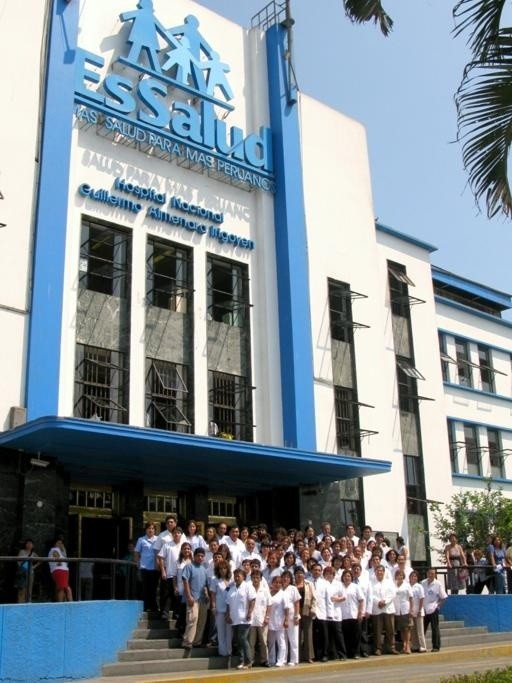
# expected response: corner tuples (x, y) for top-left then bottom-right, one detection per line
(181, 640), (202, 649)
(205, 640), (219, 649)
(236, 647), (439, 671)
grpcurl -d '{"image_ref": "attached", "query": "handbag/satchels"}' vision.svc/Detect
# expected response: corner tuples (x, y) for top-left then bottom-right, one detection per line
(13, 565), (28, 590)
(458, 565), (469, 585)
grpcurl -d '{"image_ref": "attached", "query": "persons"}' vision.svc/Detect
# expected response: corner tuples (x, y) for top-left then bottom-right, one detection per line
(77, 545), (97, 600)
(460, 533), (512, 594)
(14, 537), (44, 603)
(49, 538), (75, 603)
(444, 534), (469, 593)
(120, 539), (145, 602)
